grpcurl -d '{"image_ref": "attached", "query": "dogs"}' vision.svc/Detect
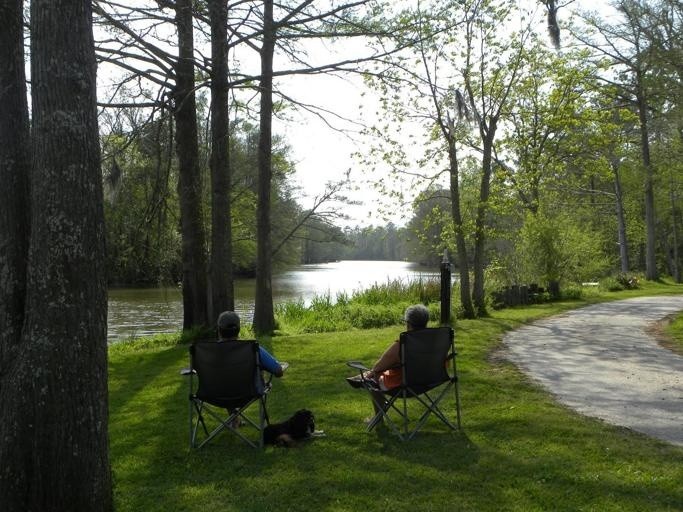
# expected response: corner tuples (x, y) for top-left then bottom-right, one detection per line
(263, 409), (328, 448)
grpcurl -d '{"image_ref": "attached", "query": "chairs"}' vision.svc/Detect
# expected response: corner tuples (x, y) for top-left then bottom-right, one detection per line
(344, 326), (461, 444)
(179, 338), (290, 453)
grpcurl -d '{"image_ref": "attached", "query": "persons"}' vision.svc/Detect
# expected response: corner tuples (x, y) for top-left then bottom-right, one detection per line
(347, 304), (450, 423)
(215, 310), (284, 429)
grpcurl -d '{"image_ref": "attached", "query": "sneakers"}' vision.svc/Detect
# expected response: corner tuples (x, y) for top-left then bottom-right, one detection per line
(345, 371), (378, 389)
